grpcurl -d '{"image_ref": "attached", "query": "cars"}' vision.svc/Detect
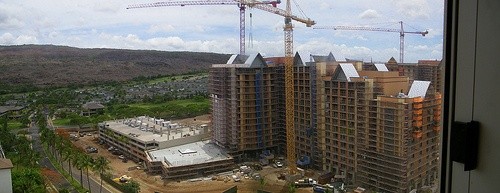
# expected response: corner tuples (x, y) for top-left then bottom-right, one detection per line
(118, 155), (126, 159)
(79, 132), (92, 137)
(232, 175), (240, 181)
(86, 148), (98, 153)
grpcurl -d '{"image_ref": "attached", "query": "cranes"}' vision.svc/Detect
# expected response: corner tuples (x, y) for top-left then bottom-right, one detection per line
(236, 0), (316, 176)
(127, 0), (282, 64)
(313, 20), (428, 77)
